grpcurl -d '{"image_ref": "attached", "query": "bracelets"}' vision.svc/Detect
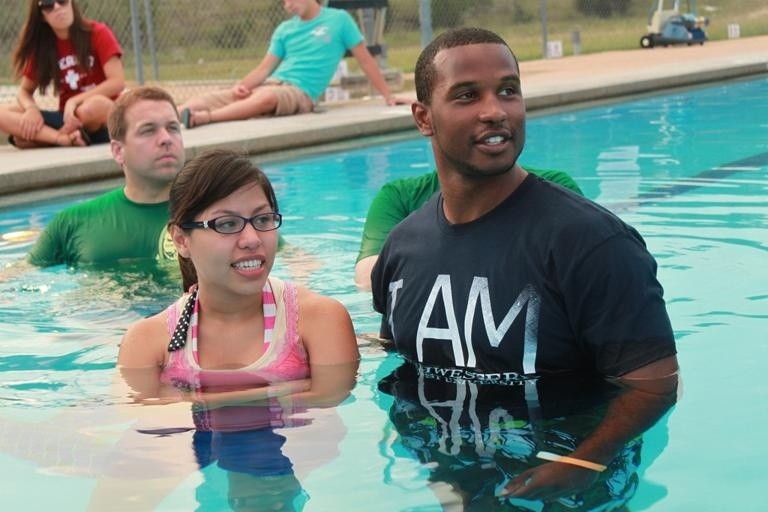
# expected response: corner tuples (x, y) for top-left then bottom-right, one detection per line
(24, 104), (41, 111)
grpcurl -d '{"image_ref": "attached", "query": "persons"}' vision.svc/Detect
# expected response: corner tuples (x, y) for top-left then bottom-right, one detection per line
(173, 1), (420, 131)
(0, 1), (129, 149)
(351, 163), (586, 304)
(1, 86), (324, 317)
(366, 26), (679, 511)
(72, 147), (363, 511)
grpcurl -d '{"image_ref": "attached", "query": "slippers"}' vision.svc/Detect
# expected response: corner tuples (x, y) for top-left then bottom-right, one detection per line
(68, 127), (92, 147)
(180, 108), (196, 129)
(7, 133), (21, 149)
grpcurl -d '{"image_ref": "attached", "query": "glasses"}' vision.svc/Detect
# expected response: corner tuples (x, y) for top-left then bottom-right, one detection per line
(179, 211), (283, 236)
(38, 0), (70, 14)
